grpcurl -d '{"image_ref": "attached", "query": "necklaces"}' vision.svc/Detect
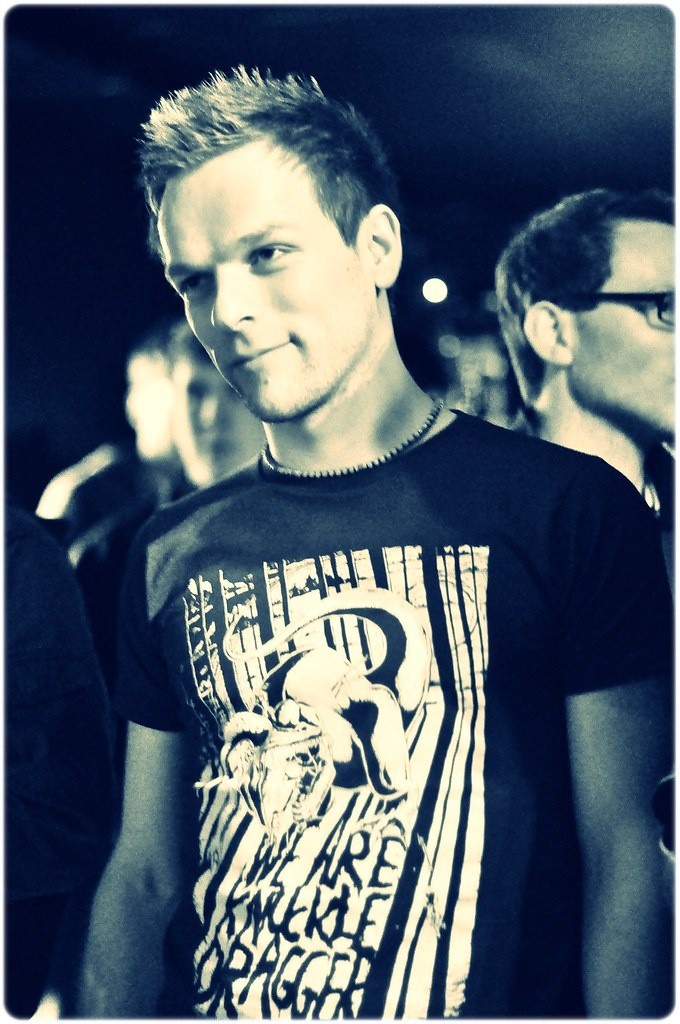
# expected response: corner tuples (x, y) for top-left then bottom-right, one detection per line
(260, 396), (444, 478)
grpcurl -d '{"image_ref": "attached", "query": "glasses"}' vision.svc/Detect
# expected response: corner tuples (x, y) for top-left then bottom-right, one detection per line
(548, 291), (675, 326)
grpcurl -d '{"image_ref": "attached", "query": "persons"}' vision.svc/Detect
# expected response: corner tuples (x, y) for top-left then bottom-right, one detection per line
(32, 320), (268, 691)
(494, 186), (674, 582)
(5, 507), (115, 1019)
(77, 67), (674, 1020)
